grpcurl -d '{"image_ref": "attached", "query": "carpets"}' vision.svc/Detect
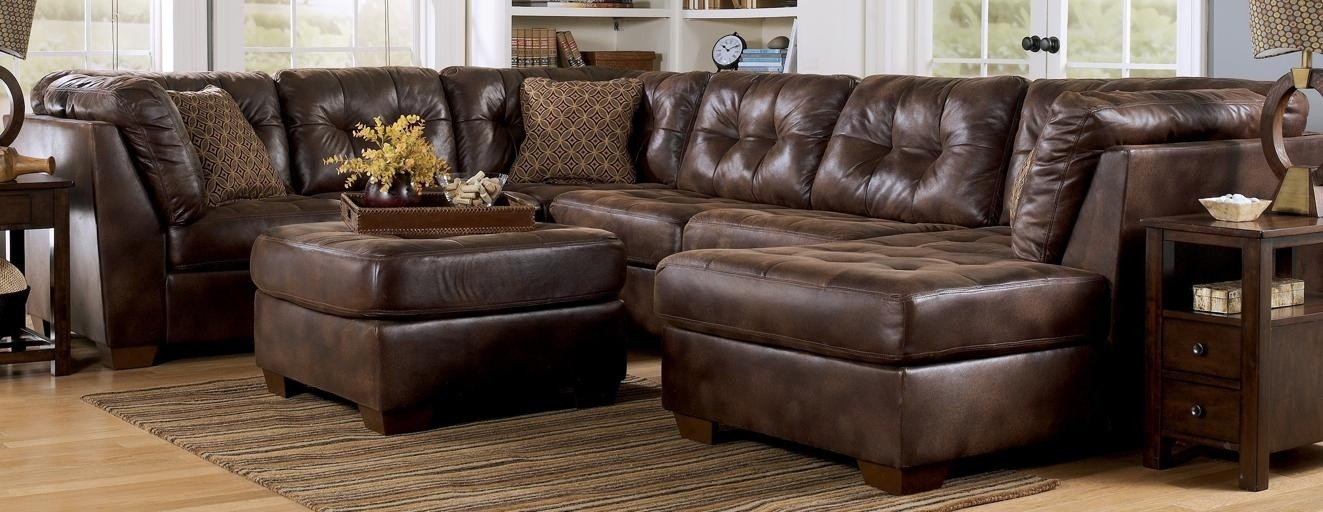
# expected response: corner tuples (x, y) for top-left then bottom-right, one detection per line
(78, 370), (1063, 512)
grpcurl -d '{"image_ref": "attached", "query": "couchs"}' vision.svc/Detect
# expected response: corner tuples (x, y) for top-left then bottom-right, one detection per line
(3, 66), (1322, 493)
(250, 220), (631, 436)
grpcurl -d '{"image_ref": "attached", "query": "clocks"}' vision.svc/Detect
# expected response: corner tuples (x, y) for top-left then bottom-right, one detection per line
(711, 30), (748, 73)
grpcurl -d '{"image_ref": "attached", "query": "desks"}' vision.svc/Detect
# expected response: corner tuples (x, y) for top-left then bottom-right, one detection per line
(1, 169), (76, 377)
(1137, 212), (1323, 491)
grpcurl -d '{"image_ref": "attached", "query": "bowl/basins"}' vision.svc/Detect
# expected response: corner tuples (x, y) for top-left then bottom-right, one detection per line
(433, 170), (510, 207)
(1198, 198), (1272, 221)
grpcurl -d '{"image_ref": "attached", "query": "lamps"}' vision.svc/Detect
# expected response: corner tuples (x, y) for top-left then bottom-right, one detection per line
(1246, 1), (1322, 221)
(0, 1), (39, 147)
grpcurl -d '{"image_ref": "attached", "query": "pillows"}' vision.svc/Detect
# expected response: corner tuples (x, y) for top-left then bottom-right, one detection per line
(505, 75), (646, 187)
(164, 82), (291, 210)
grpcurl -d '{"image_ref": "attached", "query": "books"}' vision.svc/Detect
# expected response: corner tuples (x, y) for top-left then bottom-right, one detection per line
(683, 0), (796, 10)
(511, 27), (587, 68)
(736, 47), (788, 73)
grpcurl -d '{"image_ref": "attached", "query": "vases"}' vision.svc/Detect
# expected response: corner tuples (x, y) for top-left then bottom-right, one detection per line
(365, 170), (423, 206)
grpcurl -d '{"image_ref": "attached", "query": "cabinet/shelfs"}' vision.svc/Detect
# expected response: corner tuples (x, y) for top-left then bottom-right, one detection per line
(465, 0), (866, 78)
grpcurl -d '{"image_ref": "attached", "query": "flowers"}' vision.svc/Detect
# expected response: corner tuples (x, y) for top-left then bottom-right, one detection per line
(320, 111), (452, 196)
